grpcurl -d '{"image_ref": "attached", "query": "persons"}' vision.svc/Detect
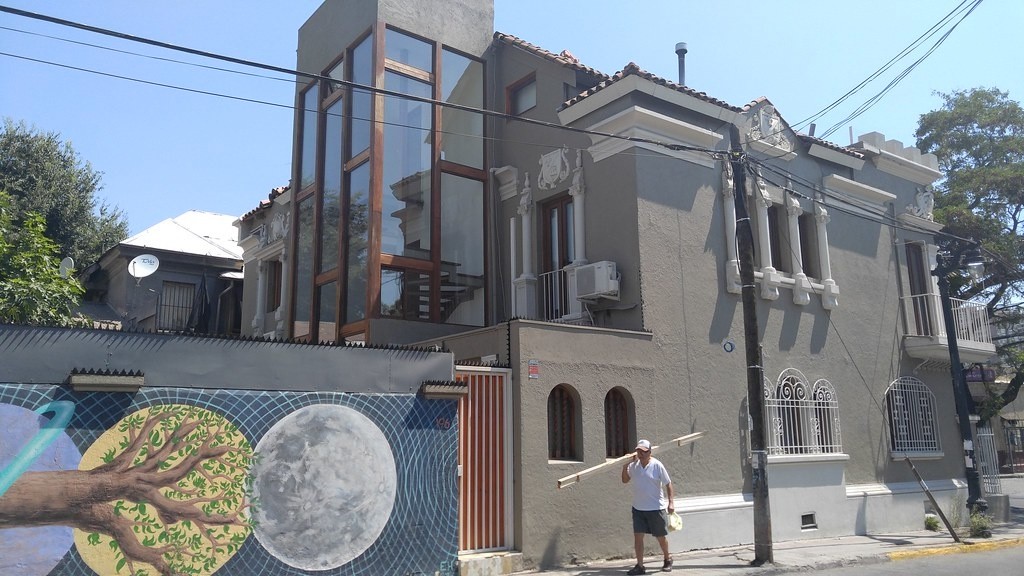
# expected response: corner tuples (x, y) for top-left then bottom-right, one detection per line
(621, 440), (675, 576)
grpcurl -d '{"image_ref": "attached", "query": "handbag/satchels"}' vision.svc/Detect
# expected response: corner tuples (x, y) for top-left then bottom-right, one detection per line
(667, 511), (682, 532)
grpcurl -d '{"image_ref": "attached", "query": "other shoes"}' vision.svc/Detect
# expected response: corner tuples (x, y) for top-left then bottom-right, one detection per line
(662, 553), (673, 571)
(627, 564), (645, 575)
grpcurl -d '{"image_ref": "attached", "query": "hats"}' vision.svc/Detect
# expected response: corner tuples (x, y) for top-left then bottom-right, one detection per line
(635, 440), (651, 452)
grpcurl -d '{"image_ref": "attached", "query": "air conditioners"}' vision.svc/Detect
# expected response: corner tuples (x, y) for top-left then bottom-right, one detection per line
(575, 261), (618, 299)
